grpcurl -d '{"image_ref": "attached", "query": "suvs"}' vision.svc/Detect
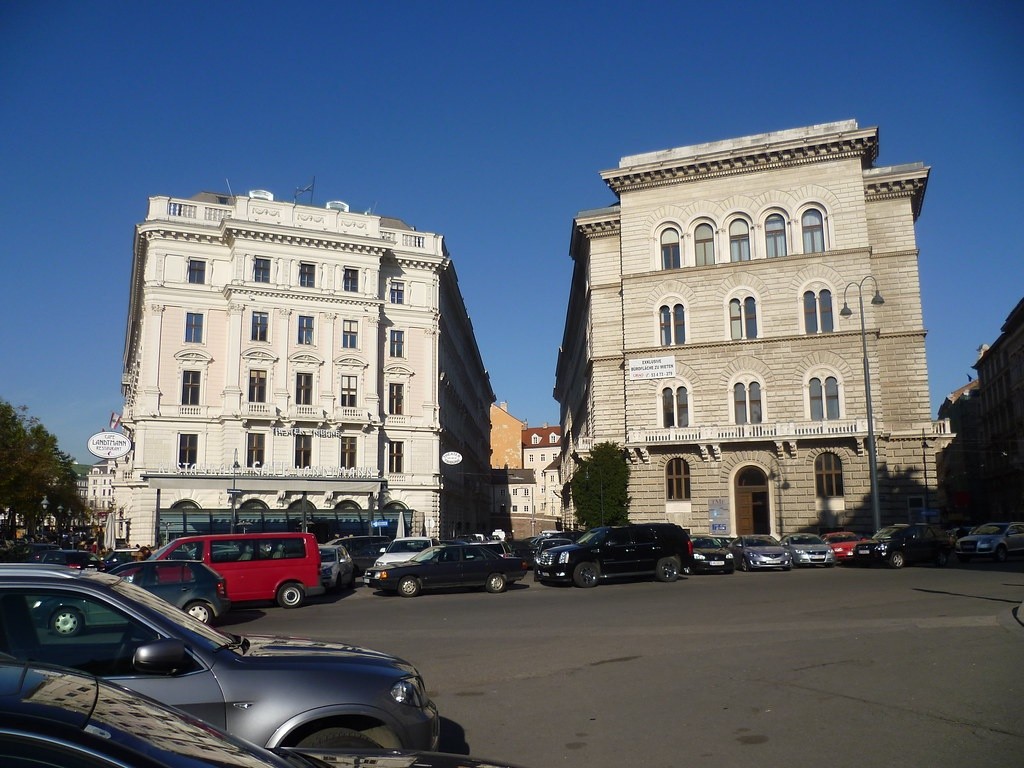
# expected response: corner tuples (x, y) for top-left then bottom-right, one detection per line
(374, 536), (440, 568)
(101, 549), (145, 572)
(538, 522), (695, 588)
(853, 524), (953, 569)
(32, 561), (231, 638)
(0, 562), (441, 750)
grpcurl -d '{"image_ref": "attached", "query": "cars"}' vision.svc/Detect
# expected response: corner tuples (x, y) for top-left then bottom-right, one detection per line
(441, 533), (586, 582)
(317, 544), (355, 600)
(778, 533), (836, 569)
(361, 544), (527, 597)
(29, 548), (105, 574)
(20, 544), (62, 563)
(727, 534), (793, 572)
(946, 522), (1024, 564)
(688, 535), (735, 575)
(820, 532), (866, 565)
(0, 659), (524, 768)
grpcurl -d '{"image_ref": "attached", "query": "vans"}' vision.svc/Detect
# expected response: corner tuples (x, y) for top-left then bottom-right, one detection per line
(320, 535), (392, 572)
(117, 532), (326, 609)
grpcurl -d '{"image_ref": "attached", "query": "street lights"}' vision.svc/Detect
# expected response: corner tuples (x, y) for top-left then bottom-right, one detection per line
(230, 448), (240, 533)
(839, 275), (885, 536)
(919, 428), (929, 524)
